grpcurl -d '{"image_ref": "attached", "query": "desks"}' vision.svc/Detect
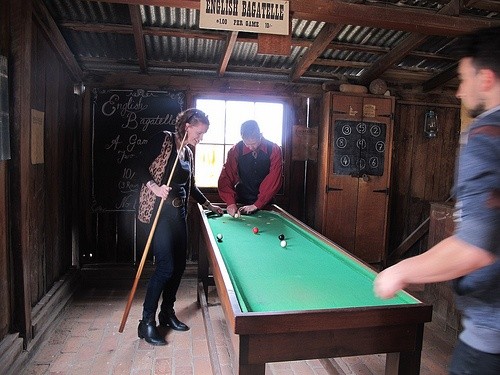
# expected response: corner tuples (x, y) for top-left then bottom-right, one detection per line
(195, 200), (433, 375)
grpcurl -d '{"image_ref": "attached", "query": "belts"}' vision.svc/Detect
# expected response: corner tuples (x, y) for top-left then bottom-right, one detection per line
(165, 197), (191, 209)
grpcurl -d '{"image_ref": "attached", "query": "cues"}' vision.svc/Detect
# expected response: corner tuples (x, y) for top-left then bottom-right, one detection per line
(118, 130), (189, 334)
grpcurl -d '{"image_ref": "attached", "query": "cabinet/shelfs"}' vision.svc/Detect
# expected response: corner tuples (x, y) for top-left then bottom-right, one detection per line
(314, 91), (395, 267)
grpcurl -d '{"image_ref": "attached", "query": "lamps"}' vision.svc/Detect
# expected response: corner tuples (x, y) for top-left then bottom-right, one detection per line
(424, 110), (439, 138)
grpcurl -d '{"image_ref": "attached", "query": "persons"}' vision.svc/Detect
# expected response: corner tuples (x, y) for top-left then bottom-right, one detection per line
(375, 25), (500, 375)
(135, 111), (222, 346)
(217, 120), (283, 218)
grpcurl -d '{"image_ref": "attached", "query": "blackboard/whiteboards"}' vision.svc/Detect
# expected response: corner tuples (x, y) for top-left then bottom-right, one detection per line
(90, 89), (190, 212)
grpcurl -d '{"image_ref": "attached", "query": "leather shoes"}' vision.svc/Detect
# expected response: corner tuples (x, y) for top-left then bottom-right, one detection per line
(158, 311), (190, 332)
(137, 320), (167, 347)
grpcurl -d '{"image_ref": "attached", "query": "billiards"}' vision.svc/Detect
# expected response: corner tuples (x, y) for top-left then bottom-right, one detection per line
(253, 227), (259, 233)
(218, 234), (223, 239)
(280, 241), (287, 248)
(234, 214), (239, 219)
(279, 235), (284, 240)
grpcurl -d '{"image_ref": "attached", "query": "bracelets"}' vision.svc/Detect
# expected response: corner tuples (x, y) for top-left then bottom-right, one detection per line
(203, 200), (210, 208)
